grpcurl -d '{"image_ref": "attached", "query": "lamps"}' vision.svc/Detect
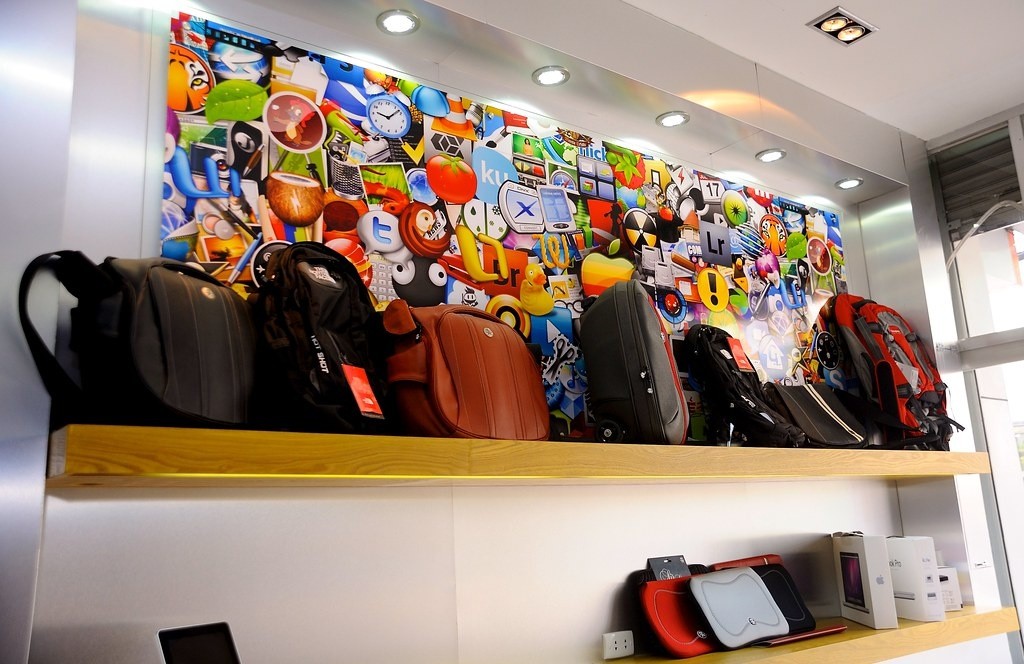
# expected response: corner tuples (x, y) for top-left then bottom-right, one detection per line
(834, 178), (864, 191)
(532, 66), (571, 88)
(805, 6), (881, 48)
(655, 111), (690, 128)
(755, 148), (786, 163)
(376, 10), (420, 37)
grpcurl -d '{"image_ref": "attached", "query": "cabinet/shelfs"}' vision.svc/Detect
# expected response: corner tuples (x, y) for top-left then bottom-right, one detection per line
(46, 426), (1023, 664)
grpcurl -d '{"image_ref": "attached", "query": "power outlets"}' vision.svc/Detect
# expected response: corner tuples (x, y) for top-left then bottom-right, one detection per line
(602, 630), (634, 659)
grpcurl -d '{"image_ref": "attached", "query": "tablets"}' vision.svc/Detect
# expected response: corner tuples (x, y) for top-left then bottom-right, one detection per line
(157, 622), (242, 664)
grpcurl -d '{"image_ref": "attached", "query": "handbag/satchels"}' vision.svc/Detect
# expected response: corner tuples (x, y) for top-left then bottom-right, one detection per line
(384, 298), (551, 440)
(17, 250), (251, 421)
(765, 372), (865, 449)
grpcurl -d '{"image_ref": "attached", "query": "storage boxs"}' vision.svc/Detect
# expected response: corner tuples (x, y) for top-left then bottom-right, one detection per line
(938, 566), (963, 611)
(831, 531), (898, 629)
(885, 535), (945, 622)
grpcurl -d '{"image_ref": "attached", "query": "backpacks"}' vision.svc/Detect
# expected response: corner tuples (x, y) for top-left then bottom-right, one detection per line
(581, 275), (687, 443)
(248, 243), (392, 432)
(815, 290), (964, 448)
(688, 322), (808, 446)
